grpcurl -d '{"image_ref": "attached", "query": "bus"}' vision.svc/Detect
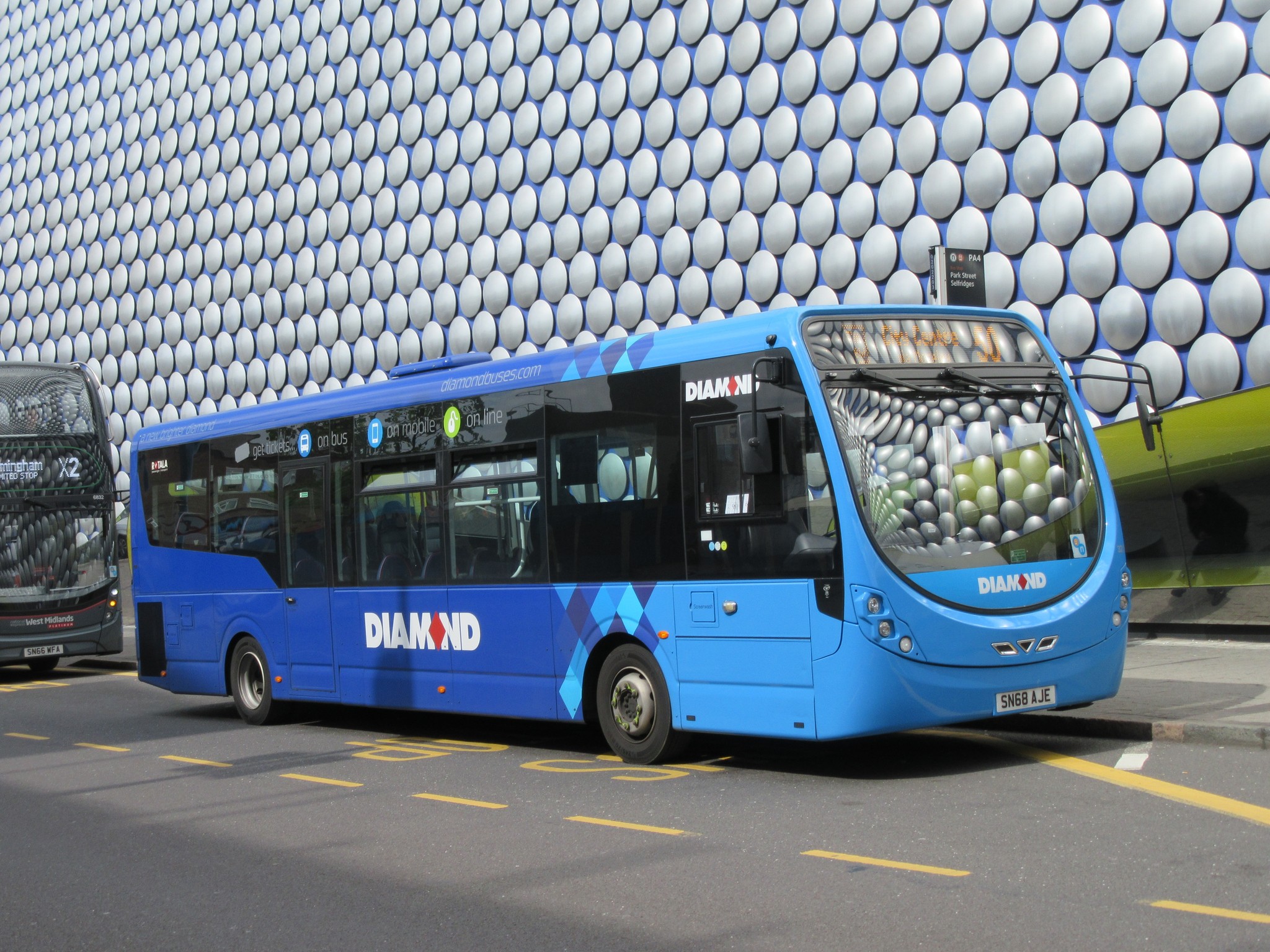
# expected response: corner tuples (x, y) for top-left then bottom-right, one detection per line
(127, 306), (1163, 764)
(0, 360), (124, 674)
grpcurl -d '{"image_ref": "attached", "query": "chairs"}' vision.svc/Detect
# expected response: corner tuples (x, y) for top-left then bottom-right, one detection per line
(203, 493), (577, 582)
